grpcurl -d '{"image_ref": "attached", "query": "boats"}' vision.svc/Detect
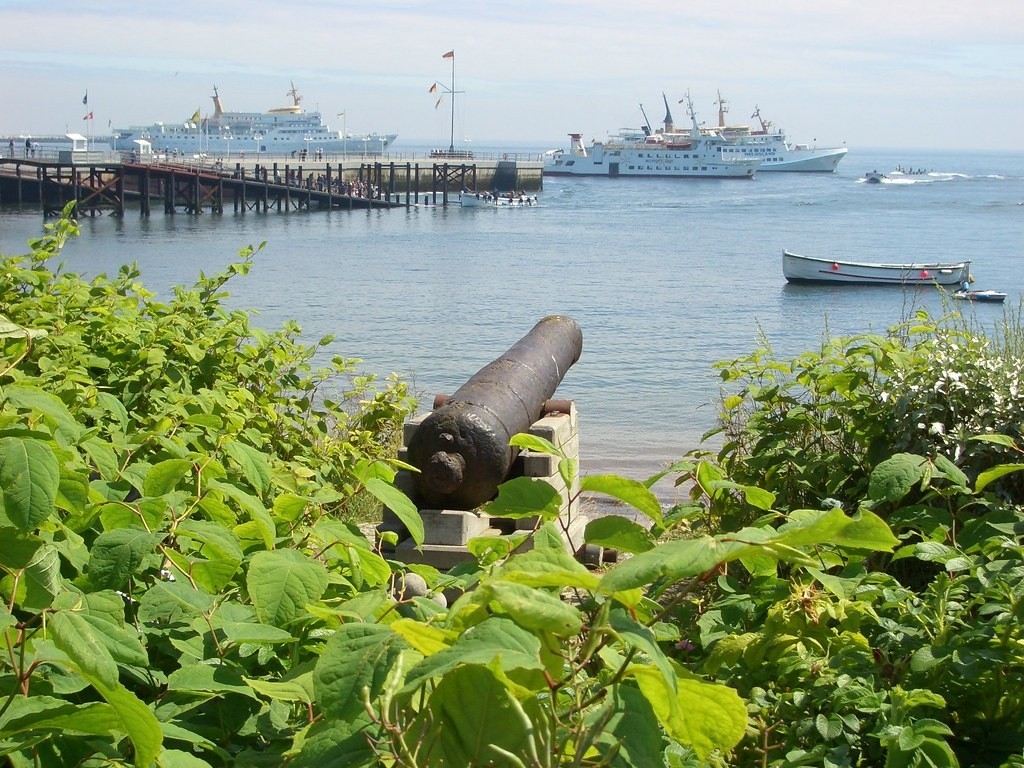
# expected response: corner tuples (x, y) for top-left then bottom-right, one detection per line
(666, 140), (692, 149)
(542, 90), (766, 180)
(109, 81), (399, 158)
(950, 282), (1008, 301)
(782, 247), (975, 286)
(614, 87), (848, 173)
(864, 168), (888, 183)
(895, 163), (930, 181)
(460, 189), (539, 208)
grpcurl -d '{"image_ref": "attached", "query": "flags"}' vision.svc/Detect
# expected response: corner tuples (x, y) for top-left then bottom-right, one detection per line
(435, 96), (443, 108)
(83, 96), (87, 104)
(443, 51), (454, 58)
(191, 110), (200, 122)
(202, 118), (206, 129)
(430, 83), (437, 91)
(84, 113), (92, 120)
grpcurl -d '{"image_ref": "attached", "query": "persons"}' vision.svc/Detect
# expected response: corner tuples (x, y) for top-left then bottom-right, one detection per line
(275, 166), (379, 199)
(165, 146), (170, 162)
(292, 149), (297, 158)
(493, 186), (526, 200)
(31, 141), (40, 156)
(203, 151), (211, 158)
(10, 139), (16, 158)
(25, 137), (32, 156)
(301, 149), (308, 162)
(463, 184), (470, 193)
(173, 148), (178, 158)
(240, 150), (245, 161)
(314, 147), (323, 161)
(130, 148), (136, 163)
(181, 150), (185, 157)
(899, 164), (926, 173)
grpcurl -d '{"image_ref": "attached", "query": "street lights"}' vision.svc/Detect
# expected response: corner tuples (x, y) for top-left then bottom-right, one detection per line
(114, 131), (119, 161)
(224, 132), (233, 158)
(361, 134), (371, 160)
(464, 139), (472, 159)
(254, 134), (263, 160)
(379, 136), (388, 159)
(303, 133), (314, 156)
